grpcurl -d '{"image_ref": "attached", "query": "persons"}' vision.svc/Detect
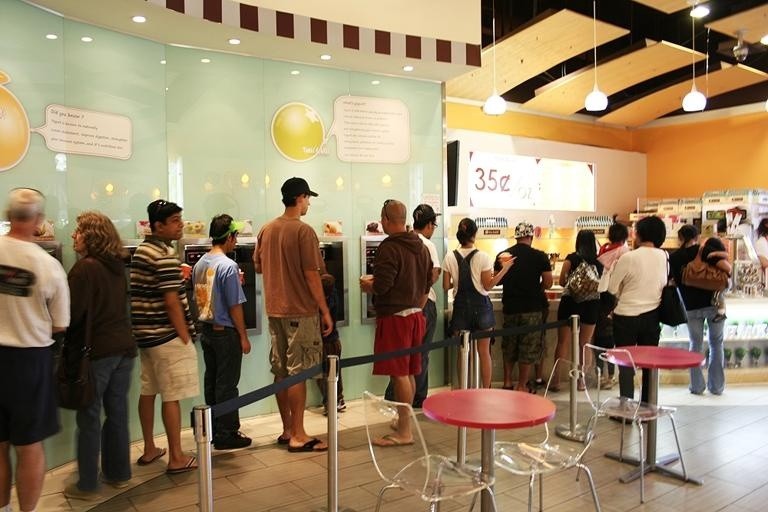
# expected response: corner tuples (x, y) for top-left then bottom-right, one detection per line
(443, 218), (516, 388)
(64, 212), (139, 501)
(591, 223), (630, 293)
(253, 176), (328, 452)
(605, 215), (670, 425)
(315, 271), (346, 416)
(130, 199), (200, 474)
(593, 292), (618, 389)
(370, 200), (434, 447)
(0, 189), (71, 511)
(546, 230), (604, 392)
(670, 225), (732, 394)
(702, 237), (729, 323)
(192, 213), (252, 451)
(755, 218), (768, 268)
(493, 222), (553, 395)
(385, 203), (442, 409)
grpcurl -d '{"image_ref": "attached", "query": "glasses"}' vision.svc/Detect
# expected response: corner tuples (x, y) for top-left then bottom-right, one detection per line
(383, 199), (394, 221)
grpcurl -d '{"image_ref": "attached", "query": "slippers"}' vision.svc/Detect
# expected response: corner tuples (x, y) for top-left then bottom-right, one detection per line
(288, 438), (328, 451)
(372, 434), (415, 447)
(390, 420), (401, 431)
(166, 456), (199, 474)
(277, 433), (290, 444)
(137, 448), (165, 465)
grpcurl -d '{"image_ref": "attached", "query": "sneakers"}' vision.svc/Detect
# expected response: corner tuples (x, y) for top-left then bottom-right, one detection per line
(323, 405), (328, 415)
(544, 381), (561, 392)
(214, 433), (251, 449)
(64, 483), (102, 501)
(712, 313), (726, 323)
(99, 471), (128, 488)
(336, 398), (347, 410)
(535, 377), (544, 389)
(600, 376), (617, 390)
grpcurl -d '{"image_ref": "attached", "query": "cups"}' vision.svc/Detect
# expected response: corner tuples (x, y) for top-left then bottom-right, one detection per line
(500, 255), (512, 266)
(239, 272), (244, 284)
(548, 293), (555, 299)
(360, 275), (374, 292)
(180, 266), (192, 279)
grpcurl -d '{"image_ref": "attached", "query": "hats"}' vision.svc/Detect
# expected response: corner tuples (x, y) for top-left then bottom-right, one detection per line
(280, 177), (319, 198)
(457, 218), (477, 244)
(146, 199), (183, 223)
(413, 204), (442, 225)
(514, 222), (534, 239)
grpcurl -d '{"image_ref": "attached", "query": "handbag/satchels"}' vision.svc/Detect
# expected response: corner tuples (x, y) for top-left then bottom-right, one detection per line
(54, 290), (97, 409)
(565, 261), (600, 303)
(681, 238), (729, 291)
(660, 250), (688, 327)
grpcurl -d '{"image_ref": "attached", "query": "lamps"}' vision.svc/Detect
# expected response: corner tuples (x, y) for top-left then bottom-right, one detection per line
(682, 1), (706, 112)
(761, 34), (768, 49)
(688, 4), (712, 20)
(483, 1), (507, 116)
(584, 0), (608, 112)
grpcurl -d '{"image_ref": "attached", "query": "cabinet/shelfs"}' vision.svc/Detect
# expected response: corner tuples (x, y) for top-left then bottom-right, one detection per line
(448, 286), (564, 384)
(657, 287), (768, 385)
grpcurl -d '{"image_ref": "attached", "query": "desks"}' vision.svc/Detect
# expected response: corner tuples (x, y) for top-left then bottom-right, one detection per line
(422, 388), (556, 512)
(604, 346), (707, 488)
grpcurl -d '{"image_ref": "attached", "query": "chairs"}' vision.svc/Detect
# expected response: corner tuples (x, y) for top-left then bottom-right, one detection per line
(576, 343), (688, 504)
(469, 358), (601, 512)
(361, 390), (499, 512)
